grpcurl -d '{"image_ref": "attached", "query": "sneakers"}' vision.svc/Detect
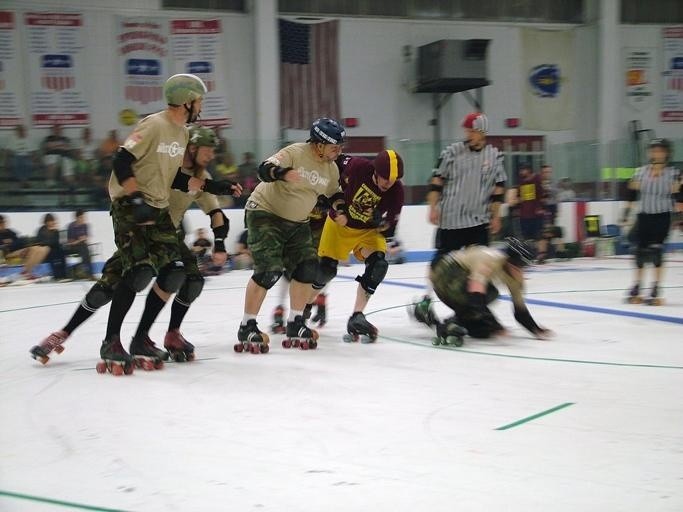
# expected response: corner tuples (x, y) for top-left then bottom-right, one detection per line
(534, 248), (570, 265)
(56, 275), (78, 283)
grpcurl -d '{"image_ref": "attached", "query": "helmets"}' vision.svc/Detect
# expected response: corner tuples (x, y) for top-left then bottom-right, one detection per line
(187, 123), (221, 148)
(373, 149), (403, 181)
(497, 236), (541, 267)
(309, 115), (347, 146)
(643, 137), (674, 161)
(163, 72), (209, 107)
(460, 112), (491, 134)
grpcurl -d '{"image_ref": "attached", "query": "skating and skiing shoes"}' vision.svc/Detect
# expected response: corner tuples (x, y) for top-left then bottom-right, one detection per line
(129, 332), (169, 371)
(233, 319), (270, 354)
(96, 337), (137, 375)
(30, 331), (67, 364)
(344, 311), (379, 345)
(414, 298), (437, 328)
(432, 321), (468, 346)
(164, 329), (195, 362)
(272, 294), (328, 351)
(625, 282), (666, 307)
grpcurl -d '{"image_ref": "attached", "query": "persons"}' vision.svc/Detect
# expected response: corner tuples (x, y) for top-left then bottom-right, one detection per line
(271, 139), (327, 328)
(302, 148), (405, 335)
(187, 221), (260, 274)
(29, 122), (230, 358)
(236, 116), (350, 344)
(426, 110), (508, 255)
(0, 122), (262, 207)
(99, 73), (244, 363)
(415, 239), (555, 339)
(506, 135), (683, 297)
(1, 212), (99, 286)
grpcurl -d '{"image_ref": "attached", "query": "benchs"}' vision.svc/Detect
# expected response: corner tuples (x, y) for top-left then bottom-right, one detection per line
(0, 146), (99, 211)
(0, 262), (53, 280)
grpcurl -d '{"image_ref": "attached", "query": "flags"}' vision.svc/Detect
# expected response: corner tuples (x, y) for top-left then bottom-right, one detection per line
(278, 17), (341, 132)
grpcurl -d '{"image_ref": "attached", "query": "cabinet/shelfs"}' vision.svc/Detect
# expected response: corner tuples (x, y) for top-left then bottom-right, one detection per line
(484, 136), (548, 191)
(339, 136), (387, 157)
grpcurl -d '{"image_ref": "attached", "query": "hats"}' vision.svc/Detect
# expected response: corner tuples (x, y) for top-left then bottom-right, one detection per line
(44, 213), (57, 223)
